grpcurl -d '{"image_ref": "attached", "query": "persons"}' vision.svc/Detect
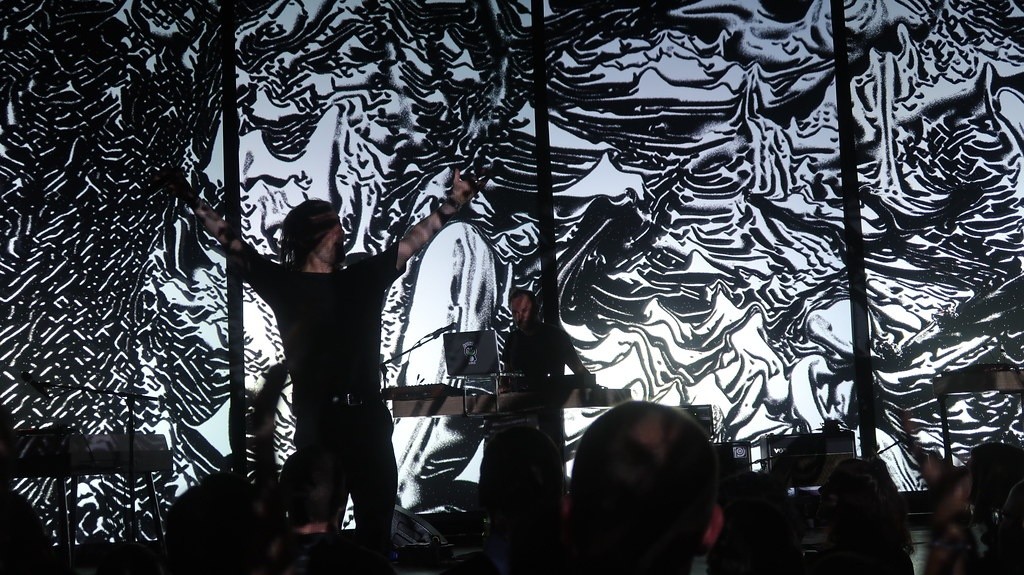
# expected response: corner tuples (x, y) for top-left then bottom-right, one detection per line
(145, 161), (492, 555)
(1, 404), (1024, 575)
(501, 290), (582, 460)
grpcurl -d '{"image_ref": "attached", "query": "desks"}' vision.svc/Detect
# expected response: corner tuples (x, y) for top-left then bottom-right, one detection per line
(390, 388), (633, 489)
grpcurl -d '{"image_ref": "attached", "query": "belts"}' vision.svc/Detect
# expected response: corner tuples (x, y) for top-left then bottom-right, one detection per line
(319, 393), (371, 407)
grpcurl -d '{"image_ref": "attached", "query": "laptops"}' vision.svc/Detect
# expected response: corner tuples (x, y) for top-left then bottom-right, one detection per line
(443, 331), (525, 377)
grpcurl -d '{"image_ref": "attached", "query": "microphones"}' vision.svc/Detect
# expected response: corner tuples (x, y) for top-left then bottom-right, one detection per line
(424, 322), (458, 339)
(21, 372), (51, 401)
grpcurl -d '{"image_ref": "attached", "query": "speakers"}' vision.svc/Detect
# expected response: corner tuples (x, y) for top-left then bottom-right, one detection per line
(761, 431), (856, 496)
(713, 439), (752, 508)
(968, 443), (1024, 527)
(392, 504), (455, 567)
